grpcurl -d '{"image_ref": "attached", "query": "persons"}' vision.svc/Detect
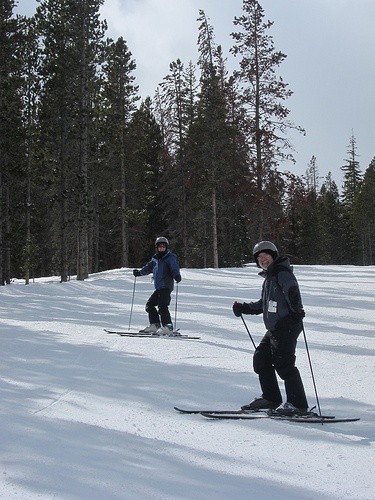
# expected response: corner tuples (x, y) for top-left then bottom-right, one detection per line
(132, 237), (182, 336)
(232, 241), (309, 416)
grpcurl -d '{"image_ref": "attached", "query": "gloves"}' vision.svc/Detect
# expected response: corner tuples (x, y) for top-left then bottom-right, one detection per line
(175, 275), (181, 283)
(232, 300), (244, 317)
(133, 270), (141, 277)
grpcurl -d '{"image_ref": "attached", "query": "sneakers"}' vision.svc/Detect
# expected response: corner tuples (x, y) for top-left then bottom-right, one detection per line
(267, 402), (307, 416)
(241, 396), (282, 410)
(156, 324), (173, 335)
(139, 324), (161, 333)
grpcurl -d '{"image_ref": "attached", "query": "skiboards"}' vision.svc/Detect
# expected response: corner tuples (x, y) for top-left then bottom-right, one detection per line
(173, 406), (361, 423)
(104, 328), (201, 339)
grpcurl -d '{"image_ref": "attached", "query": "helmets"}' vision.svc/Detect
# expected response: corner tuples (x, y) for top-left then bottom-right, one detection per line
(252, 241), (279, 268)
(155, 236), (169, 245)
